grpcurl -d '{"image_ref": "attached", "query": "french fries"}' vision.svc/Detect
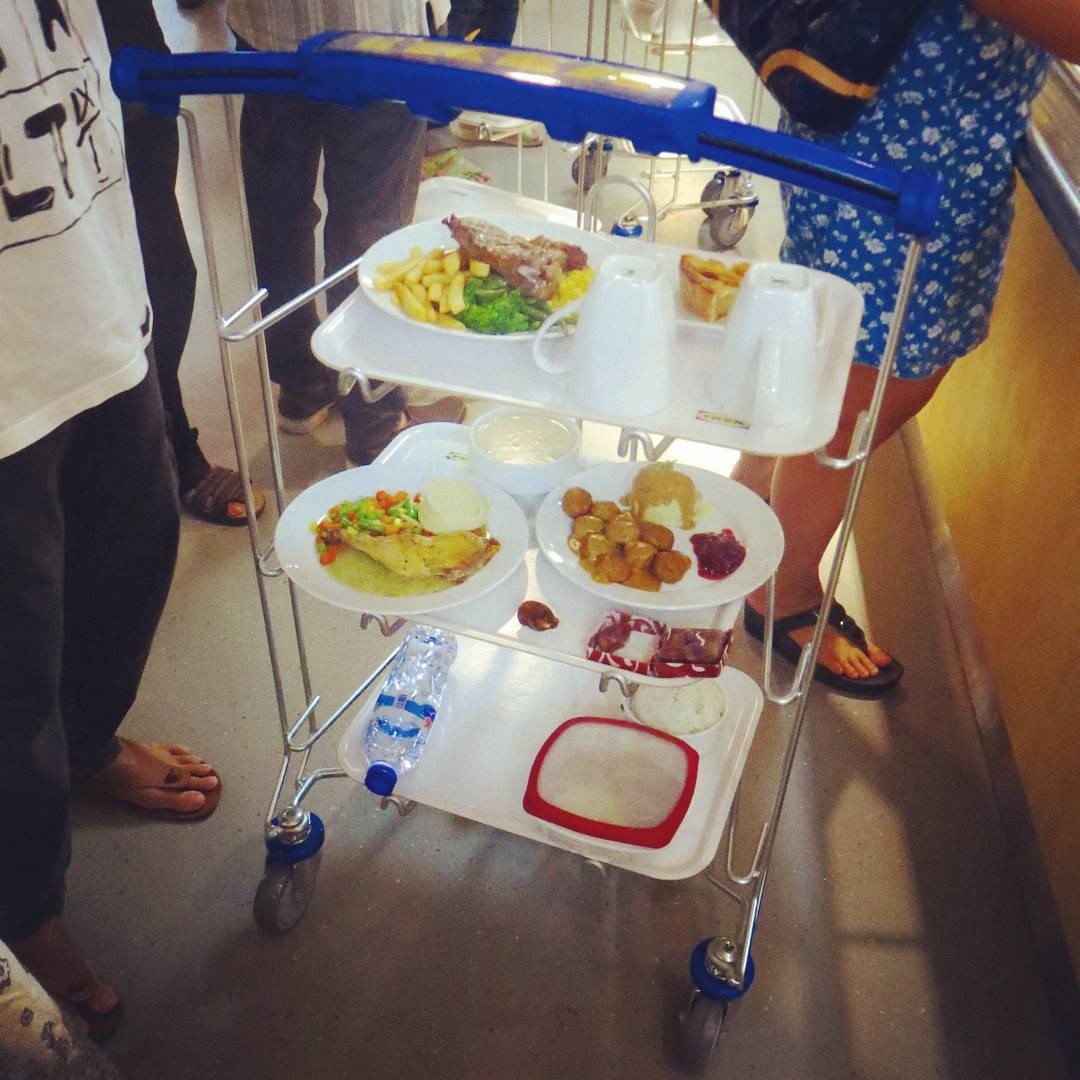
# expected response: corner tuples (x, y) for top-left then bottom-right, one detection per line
(370, 244), (486, 330)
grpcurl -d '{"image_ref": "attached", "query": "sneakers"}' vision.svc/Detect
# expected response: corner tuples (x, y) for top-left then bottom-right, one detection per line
(346, 396), (465, 469)
(279, 368), (347, 435)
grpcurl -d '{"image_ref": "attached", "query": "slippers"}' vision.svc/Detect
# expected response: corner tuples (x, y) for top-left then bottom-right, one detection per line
(67, 739), (221, 820)
(8, 925), (123, 1040)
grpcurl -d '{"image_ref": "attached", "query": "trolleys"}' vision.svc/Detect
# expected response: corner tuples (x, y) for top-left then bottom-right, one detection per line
(108, 26), (939, 1072)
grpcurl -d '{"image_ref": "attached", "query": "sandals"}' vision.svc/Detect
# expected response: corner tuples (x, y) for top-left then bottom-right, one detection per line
(178, 464), (267, 527)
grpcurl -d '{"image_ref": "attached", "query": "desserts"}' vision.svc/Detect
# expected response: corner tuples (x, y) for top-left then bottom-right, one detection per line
(677, 253), (750, 324)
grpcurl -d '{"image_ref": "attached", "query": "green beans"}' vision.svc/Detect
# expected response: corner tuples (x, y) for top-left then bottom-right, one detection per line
(329, 501), (419, 533)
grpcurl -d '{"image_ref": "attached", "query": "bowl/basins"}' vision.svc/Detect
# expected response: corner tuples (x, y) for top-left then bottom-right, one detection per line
(522, 717), (699, 849)
(623, 677), (729, 756)
(469, 404), (582, 495)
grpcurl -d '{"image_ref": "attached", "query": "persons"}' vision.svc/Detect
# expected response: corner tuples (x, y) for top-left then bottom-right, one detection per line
(97, 0), (516, 522)
(0, 0), (226, 1043)
(730, 0), (1080, 697)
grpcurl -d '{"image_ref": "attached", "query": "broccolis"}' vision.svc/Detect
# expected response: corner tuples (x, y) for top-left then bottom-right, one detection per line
(453, 279), (541, 336)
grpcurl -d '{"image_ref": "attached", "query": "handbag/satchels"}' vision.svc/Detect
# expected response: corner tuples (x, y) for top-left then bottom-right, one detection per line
(696, 0), (927, 132)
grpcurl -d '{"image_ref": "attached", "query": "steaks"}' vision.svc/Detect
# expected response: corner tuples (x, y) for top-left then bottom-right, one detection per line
(444, 213), (589, 302)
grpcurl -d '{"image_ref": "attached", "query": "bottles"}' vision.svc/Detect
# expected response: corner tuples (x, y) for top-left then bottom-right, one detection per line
(364, 623), (457, 797)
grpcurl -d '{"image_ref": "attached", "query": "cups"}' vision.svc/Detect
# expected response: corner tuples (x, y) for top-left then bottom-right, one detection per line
(711, 262), (817, 424)
(649, 0), (697, 44)
(534, 253), (675, 417)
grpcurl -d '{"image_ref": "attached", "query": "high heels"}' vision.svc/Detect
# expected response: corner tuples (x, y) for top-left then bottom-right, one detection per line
(745, 596), (904, 695)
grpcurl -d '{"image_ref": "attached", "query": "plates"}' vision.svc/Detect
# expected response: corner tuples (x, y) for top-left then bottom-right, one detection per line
(658, 94), (747, 159)
(274, 465), (529, 616)
(536, 461), (785, 609)
(664, 260), (831, 350)
(412, 176), (602, 233)
(357, 215), (619, 341)
